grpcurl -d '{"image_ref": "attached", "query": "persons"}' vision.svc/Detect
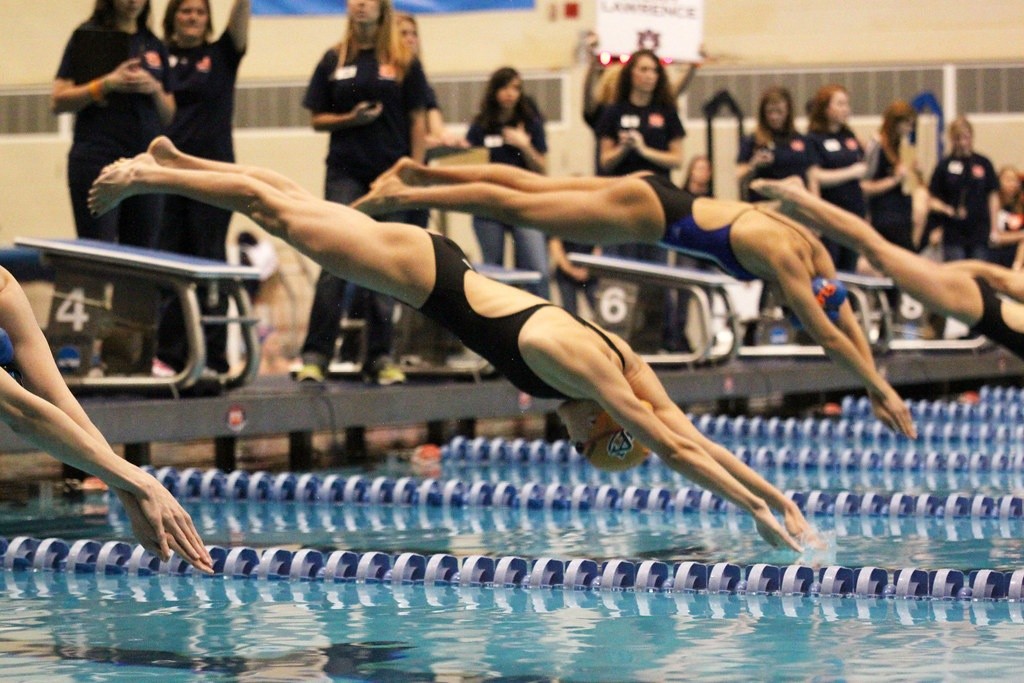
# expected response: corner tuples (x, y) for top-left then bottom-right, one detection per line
(1, 262), (216, 574)
(82, 133), (831, 550)
(46, 0), (1023, 442)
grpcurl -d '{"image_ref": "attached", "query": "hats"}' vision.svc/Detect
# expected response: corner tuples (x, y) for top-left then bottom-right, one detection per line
(582, 402), (653, 470)
(789, 277), (846, 331)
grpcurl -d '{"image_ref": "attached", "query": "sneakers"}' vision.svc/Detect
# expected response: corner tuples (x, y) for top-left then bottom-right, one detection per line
(362, 357), (405, 385)
(297, 353), (328, 382)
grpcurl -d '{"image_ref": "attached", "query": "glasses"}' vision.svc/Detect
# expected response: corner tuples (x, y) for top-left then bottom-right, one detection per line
(782, 305), (839, 319)
(576, 428), (623, 456)
